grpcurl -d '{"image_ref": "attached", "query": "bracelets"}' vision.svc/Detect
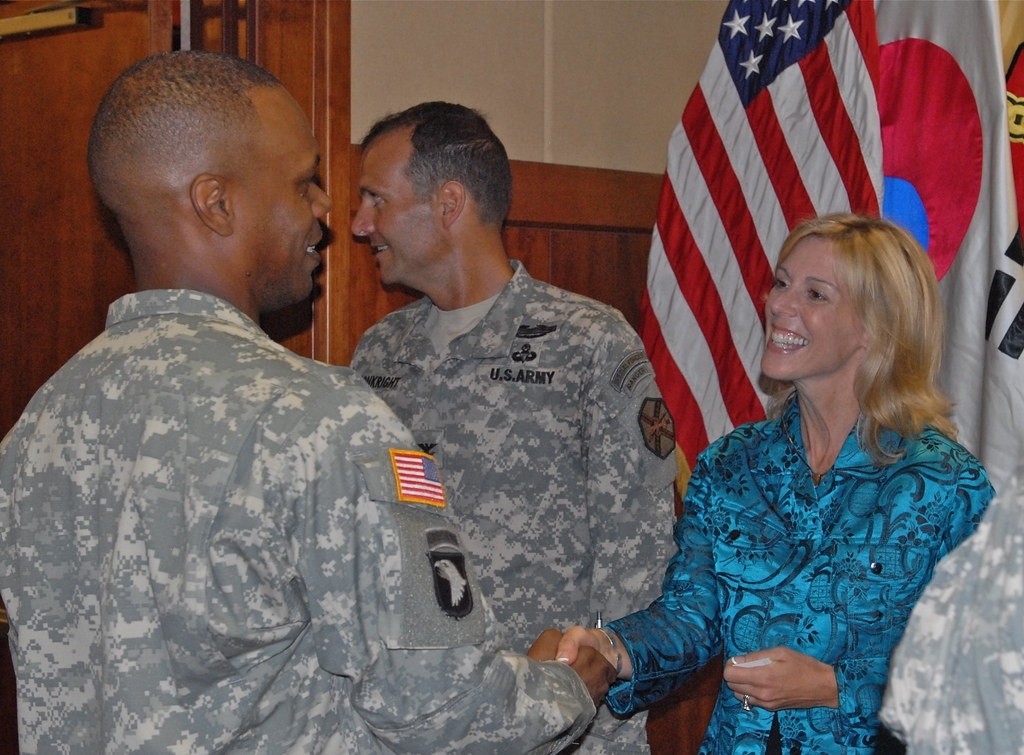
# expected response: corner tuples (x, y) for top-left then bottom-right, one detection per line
(599, 628), (623, 676)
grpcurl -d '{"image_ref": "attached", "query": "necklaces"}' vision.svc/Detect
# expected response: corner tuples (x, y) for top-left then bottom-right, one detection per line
(783, 389), (826, 484)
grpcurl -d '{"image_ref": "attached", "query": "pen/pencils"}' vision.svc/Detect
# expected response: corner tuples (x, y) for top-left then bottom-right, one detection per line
(594, 610), (603, 629)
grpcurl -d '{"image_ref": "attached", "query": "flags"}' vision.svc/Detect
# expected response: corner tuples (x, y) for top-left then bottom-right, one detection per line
(639, 0), (1024, 504)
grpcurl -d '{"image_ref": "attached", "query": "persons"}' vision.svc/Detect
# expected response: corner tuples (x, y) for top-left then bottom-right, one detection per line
(878, 461), (1024, 755)
(347, 101), (678, 755)
(557, 212), (998, 755)
(0, 50), (616, 755)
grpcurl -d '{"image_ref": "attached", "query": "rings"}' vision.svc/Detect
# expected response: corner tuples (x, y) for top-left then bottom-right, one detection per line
(743, 695), (750, 711)
